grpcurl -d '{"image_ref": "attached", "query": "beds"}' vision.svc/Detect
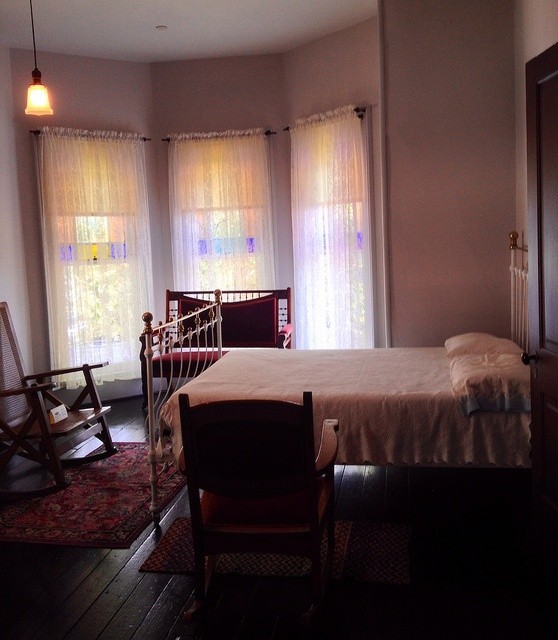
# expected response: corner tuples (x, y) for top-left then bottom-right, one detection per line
(141, 229), (534, 529)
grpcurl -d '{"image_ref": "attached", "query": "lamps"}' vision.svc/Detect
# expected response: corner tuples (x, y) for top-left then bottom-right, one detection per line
(24, 0), (54, 116)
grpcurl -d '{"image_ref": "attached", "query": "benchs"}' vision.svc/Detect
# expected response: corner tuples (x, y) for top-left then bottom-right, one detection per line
(140, 287), (292, 412)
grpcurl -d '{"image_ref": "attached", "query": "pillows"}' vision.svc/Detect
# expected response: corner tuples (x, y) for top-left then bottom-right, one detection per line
(443, 332), (532, 392)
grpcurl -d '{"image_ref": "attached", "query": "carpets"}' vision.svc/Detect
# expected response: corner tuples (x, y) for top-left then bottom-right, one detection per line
(1, 440), (187, 551)
(137, 515), (414, 586)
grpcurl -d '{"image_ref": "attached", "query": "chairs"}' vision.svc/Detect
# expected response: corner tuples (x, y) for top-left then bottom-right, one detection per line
(0, 302), (120, 501)
(178, 390), (340, 626)
